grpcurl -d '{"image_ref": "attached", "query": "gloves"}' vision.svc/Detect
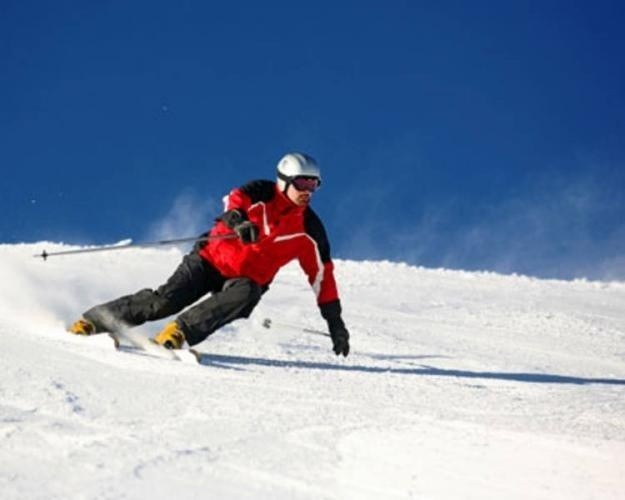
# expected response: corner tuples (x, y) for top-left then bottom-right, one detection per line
(233, 220), (259, 245)
(330, 329), (349, 356)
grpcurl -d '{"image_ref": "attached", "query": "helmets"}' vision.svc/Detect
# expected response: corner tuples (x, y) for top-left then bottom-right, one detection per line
(276, 152), (321, 191)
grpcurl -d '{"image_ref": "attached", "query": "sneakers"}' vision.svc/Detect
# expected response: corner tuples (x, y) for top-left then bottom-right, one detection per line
(155, 320), (186, 348)
(70, 318), (96, 335)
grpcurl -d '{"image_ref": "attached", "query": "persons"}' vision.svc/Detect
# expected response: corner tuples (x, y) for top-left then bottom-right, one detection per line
(70, 151), (350, 364)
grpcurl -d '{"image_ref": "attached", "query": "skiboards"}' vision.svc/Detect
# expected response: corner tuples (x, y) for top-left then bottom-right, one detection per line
(51, 330), (200, 364)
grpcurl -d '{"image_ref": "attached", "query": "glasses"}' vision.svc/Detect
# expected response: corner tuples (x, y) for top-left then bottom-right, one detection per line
(278, 172), (321, 191)
